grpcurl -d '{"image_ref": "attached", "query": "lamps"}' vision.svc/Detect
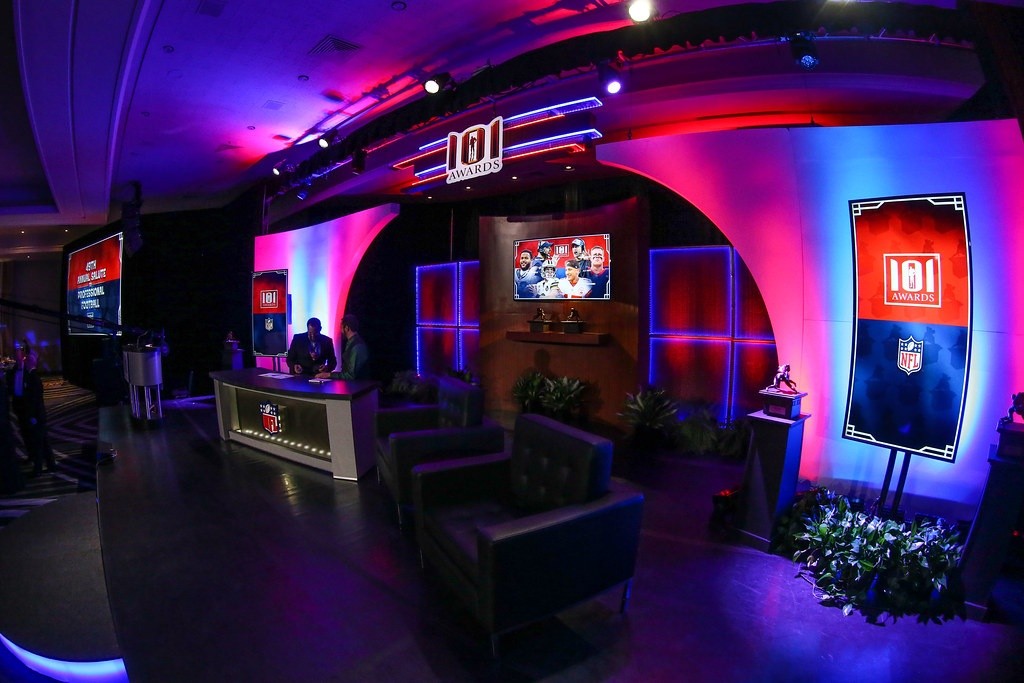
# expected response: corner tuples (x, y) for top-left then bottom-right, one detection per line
(599, 64), (623, 95)
(316, 127), (343, 149)
(789, 35), (822, 71)
(351, 149), (369, 176)
(272, 157), (298, 176)
(296, 185), (313, 201)
(421, 71), (458, 97)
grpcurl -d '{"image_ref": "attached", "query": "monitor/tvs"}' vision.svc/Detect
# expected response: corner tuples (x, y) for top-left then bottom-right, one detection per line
(513, 233), (611, 302)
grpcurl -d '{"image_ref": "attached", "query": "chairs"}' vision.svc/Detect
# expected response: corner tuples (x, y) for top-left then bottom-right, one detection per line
(409, 412), (646, 683)
(371, 374), (505, 537)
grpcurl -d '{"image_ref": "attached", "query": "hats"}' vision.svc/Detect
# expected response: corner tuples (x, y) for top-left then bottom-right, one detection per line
(538, 241), (553, 249)
(571, 238), (584, 246)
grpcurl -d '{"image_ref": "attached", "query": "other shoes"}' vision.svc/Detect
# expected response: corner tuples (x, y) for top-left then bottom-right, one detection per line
(19, 459), (32, 466)
(46, 457), (58, 471)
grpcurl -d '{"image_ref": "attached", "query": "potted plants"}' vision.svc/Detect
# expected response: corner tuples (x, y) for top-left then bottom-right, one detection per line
(614, 386), (752, 461)
(774, 484), (964, 623)
(510, 368), (598, 426)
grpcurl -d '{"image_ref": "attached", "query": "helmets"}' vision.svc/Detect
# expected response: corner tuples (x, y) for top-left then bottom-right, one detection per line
(540, 260), (557, 277)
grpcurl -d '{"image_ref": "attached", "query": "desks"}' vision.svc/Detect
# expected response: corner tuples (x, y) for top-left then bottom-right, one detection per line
(209, 367), (378, 482)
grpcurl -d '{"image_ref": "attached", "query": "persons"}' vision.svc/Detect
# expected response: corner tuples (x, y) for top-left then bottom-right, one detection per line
(532, 240), (560, 267)
(766, 364), (798, 394)
(286, 318), (337, 376)
(514, 250), (544, 298)
(551, 259), (592, 298)
(578, 246), (609, 298)
(536, 259), (563, 298)
(315, 314), (371, 381)
(4, 340), (58, 477)
(571, 238), (591, 278)
